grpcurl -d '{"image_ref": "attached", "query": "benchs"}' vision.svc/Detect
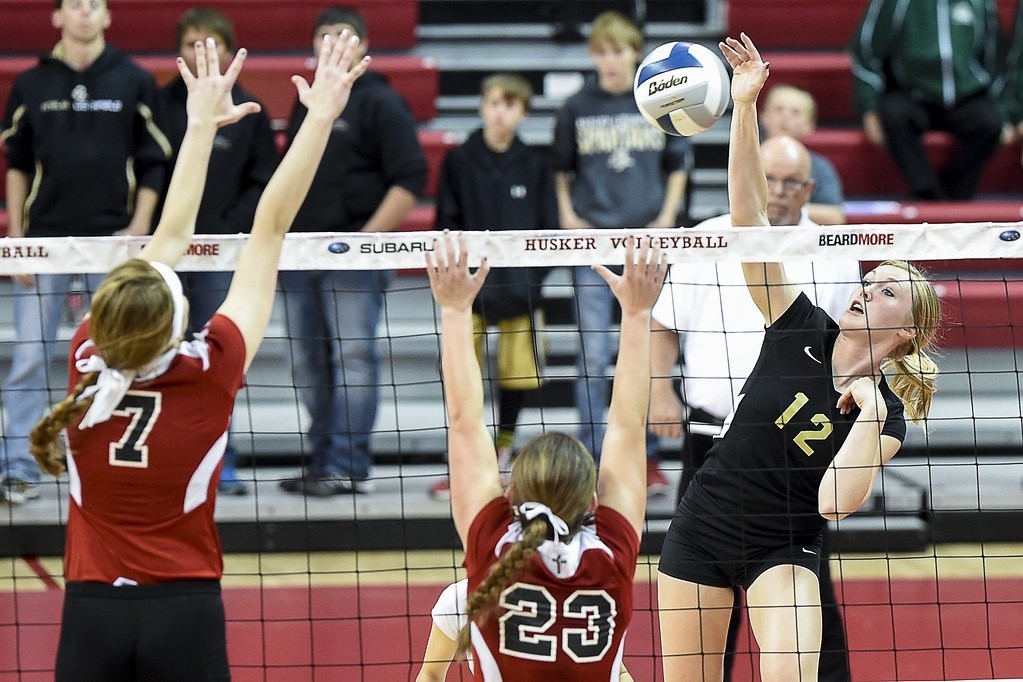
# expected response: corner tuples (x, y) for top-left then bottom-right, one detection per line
(0, 0), (463, 277)
(727, 0), (1023, 349)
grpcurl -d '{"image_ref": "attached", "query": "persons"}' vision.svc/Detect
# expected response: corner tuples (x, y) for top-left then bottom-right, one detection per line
(29, 26), (372, 682)
(422, 227), (668, 682)
(427, 73), (552, 505)
(0, 0), (164, 503)
(849, 0), (1023, 204)
(656, 32), (938, 682)
(414, 578), (635, 682)
(758, 81), (846, 225)
(154, 7), (278, 497)
(545, 9), (694, 466)
(278, 6), (425, 499)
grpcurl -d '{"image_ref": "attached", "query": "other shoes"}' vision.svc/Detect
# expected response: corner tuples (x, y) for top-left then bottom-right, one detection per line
(428, 472), (512, 501)
(279, 473), (376, 496)
(217, 468), (248, 495)
(642, 459), (671, 496)
(0, 479), (41, 505)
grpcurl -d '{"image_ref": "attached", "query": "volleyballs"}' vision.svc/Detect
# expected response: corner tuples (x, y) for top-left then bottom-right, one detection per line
(633, 42), (729, 136)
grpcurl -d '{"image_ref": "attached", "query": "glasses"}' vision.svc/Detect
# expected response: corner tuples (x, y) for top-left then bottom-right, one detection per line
(767, 172), (811, 192)
